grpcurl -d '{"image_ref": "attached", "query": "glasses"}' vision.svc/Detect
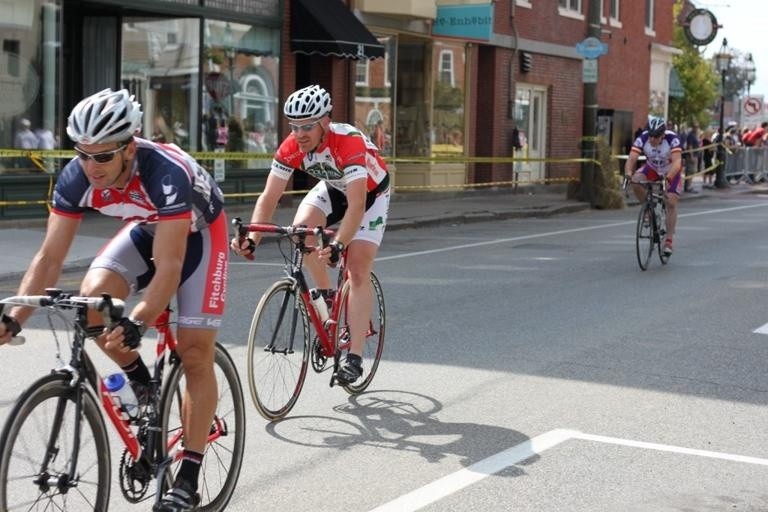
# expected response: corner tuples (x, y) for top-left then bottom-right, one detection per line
(289, 122), (319, 133)
(74, 145), (126, 164)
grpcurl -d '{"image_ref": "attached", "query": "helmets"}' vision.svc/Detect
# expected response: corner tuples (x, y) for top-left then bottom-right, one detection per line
(648, 119), (665, 136)
(66, 86), (143, 145)
(283, 85), (334, 121)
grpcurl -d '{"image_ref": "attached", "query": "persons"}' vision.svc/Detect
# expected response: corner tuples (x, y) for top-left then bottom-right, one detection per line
(153, 112), (278, 153)
(665, 120), (767, 193)
(0, 88), (230, 512)
(12, 118), (57, 166)
(355, 119), (391, 157)
(229, 85), (390, 384)
(623, 118), (685, 257)
(423, 121), (462, 146)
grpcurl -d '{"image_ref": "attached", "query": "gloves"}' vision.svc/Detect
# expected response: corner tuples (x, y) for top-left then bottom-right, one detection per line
(666, 175), (673, 185)
(2, 314), (21, 336)
(106, 318), (147, 349)
(245, 237), (256, 253)
(328, 241), (344, 262)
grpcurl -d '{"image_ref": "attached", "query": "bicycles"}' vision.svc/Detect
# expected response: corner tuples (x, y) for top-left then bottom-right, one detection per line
(0, 257), (247, 512)
(623, 169), (670, 272)
(229, 217), (386, 423)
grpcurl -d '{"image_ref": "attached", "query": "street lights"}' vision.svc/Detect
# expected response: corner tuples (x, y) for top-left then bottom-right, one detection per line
(714, 38), (733, 189)
(742, 54), (758, 97)
(221, 19), (238, 117)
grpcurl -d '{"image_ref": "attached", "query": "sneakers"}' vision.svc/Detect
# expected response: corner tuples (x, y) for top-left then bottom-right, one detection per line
(154, 479), (199, 512)
(664, 236), (672, 253)
(340, 354), (362, 383)
